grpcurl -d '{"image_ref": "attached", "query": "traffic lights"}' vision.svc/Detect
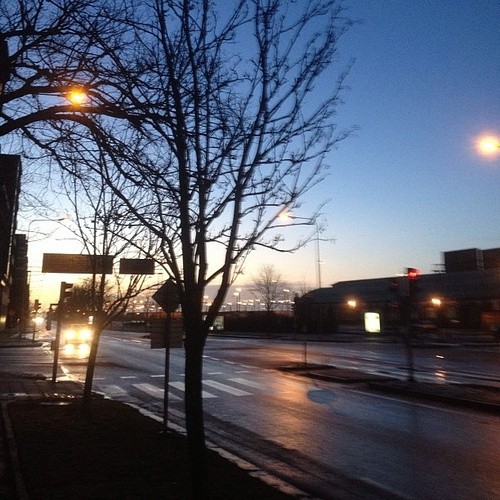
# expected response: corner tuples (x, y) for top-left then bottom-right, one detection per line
(31, 299), (41, 343)
(393, 268), (424, 379)
(44, 281), (76, 383)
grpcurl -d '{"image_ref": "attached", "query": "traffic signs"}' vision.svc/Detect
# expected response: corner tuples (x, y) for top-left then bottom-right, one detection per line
(39, 252), (114, 274)
(117, 256), (158, 276)
